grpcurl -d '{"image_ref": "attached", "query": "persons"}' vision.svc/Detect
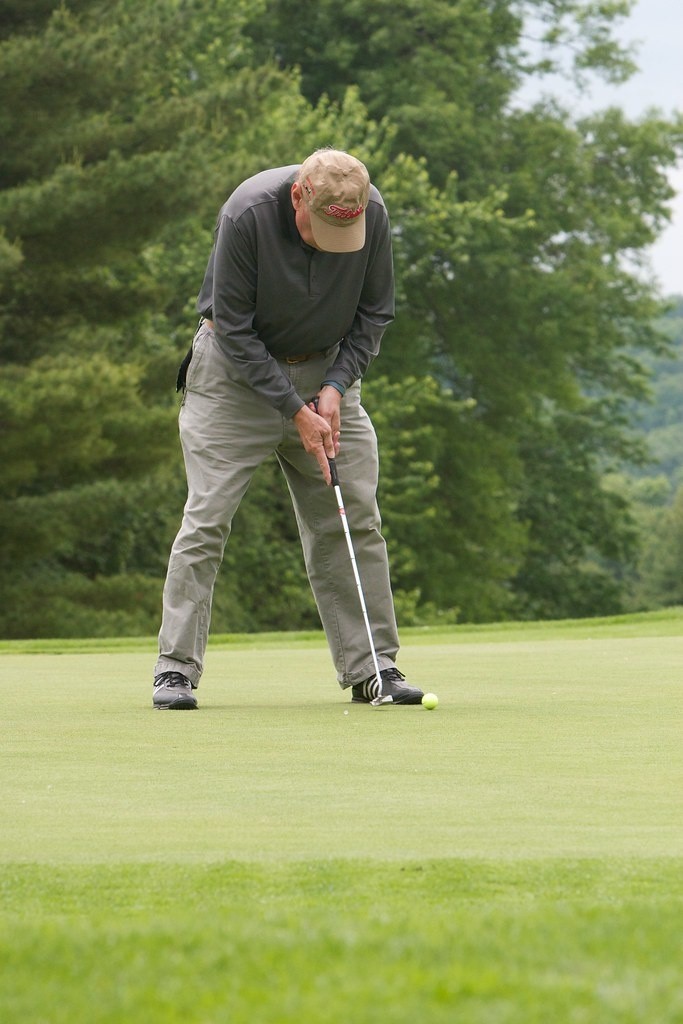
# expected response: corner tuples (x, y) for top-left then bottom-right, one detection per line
(151, 148), (425, 709)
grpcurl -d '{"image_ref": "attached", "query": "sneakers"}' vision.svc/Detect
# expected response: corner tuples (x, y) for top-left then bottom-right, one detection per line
(152, 672), (198, 710)
(352, 668), (424, 705)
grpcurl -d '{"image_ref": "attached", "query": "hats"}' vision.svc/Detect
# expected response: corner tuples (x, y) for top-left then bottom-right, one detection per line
(300, 150), (370, 253)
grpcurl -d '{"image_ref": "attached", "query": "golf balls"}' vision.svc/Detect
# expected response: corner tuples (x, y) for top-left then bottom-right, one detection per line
(422, 693), (438, 710)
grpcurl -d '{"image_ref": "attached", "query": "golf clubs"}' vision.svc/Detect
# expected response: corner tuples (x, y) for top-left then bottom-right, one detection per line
(310, 395), (395, 708)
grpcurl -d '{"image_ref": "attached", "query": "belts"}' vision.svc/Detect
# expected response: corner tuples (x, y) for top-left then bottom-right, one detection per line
(204, 317), (345, 364)
